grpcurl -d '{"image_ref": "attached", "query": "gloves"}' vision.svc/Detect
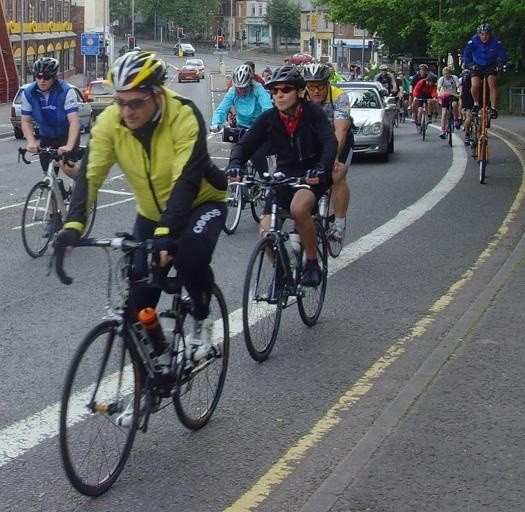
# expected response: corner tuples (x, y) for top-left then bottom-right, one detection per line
(227, 168), (245, 183)
(306, 169), (327, 185)
(152, 239), (177, 262)
(53, 228), (81, 250)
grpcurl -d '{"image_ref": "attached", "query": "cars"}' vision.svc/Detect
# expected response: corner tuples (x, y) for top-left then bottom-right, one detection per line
(81, 77), (117, 113)
(334, 82), (398, 164)
(284, 53), (315, 67)
(176, 64), (200, 83)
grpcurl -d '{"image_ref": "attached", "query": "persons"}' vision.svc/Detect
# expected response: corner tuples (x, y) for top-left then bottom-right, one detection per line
(210, 60), (353, 304)
(21, 57), (80, 238)
(374, 23), (507, 145)
(53, 51), (227, 427)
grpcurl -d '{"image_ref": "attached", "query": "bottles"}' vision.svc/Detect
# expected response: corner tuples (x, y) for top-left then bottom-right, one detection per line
(137, 306), (168, 352)
(318, 192), (328, 216)
(278, 231), (298, 268)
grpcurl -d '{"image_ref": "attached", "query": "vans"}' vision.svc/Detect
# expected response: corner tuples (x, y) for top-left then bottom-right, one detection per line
(185, 58), (204, 78)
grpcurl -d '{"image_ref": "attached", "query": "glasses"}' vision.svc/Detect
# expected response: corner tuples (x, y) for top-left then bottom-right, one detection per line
(37, 75), (51, 80)
(115, 96), (153, 107)
(270, 87), (296, 95)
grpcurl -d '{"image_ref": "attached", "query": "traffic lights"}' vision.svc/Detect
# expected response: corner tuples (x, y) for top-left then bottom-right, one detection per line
(127, 37), (135, 51)
(235, 32), (239, 40)
(177, 28), (183, 38)
(241, 30), (247, 40)
(310, 36), (314, 47)
(217, 35), (224, 49)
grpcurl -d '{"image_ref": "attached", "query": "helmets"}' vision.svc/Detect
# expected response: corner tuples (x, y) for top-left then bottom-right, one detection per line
(107, 50), (168, 91)
(419, 64), (429, 69)
(477, 23), (492, 33)
(232, 65), (254, 87)
(379, 65), (388, 69)
(32, 58), (59, 77)
(263, 53), (330, 89)
(462, 69), (470, 76)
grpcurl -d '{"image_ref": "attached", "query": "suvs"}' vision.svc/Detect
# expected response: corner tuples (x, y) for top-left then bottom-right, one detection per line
(9, 80), (94, 138)
(172, 43), (196, 56)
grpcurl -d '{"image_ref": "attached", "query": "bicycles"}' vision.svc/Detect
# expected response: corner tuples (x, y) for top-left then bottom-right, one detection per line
(15, 147), (100, 260)
(231, 166), (331, 360)
(387, 66), (505, 183)
(208, 125), (346, 258)
(46, 224), (231, 497)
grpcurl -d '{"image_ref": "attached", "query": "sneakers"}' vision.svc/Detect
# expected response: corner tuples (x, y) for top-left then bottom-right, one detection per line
(332, 218), (346, 242)
(301, 261), (322, 286)
(116, 391), (161, 428)
(185, 307), (216, 361)
(411, 106), (497, 142)
